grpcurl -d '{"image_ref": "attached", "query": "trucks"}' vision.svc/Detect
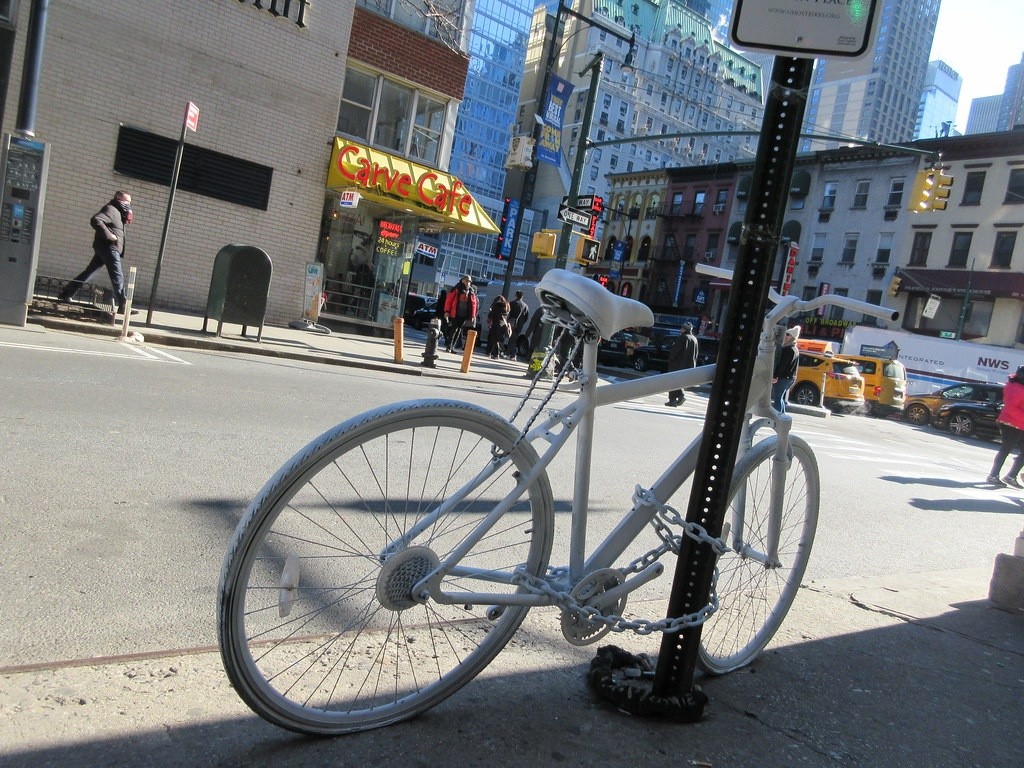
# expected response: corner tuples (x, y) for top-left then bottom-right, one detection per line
(463, 281), (541, 358)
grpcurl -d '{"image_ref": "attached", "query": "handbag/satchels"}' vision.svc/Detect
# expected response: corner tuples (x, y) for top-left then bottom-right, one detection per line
(505, 323), (513, 337)
(507, 319), (517, 329)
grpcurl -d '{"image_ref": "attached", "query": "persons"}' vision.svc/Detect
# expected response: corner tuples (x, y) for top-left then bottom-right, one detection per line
(483, 295), (511, 358)
(444, 274), (479, 354)
(770, 325), (801, 413)
(58, 190), (139, 315)
(665, 321), (699, 407)
(987, 365), (1024, 489)
(435, 290), (448, 344)
(500, 290), (528, 360)
(523, 306), (583, 376)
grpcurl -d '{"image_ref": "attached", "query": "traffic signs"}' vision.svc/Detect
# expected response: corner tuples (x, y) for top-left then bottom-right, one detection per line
(556, 204), (592, 230)
(562, 195), (595, 210)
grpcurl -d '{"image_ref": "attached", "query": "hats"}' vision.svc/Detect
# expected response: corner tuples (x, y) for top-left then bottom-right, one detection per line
(786, 325), (801, 340)
(683, 322), (693, 331)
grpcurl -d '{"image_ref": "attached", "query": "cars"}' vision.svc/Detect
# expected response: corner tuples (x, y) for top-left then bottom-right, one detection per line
(935, 402), (1002, 441)
(902, 380), (1008, 426)
(788, 350), (865, 414)
(833, 354), (907, 418)
(401, 293), (438, 331)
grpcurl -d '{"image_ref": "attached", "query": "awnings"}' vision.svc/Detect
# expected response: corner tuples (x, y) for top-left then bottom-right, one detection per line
(328, 138), (501, 235)
(898, 269), (1024, 302)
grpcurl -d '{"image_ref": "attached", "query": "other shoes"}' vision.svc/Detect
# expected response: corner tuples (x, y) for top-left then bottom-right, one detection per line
(501, 352), (508, 358)
(491, 355), (499, 359)
(118, 305), (139, 314)
(446, 348), (451, 353)
(665, 400), (677, 406)
(451, 350), (457, 354)
(677, 396), (686, 406)
(59, 291), (73, 303)
(507, 356), (516, 361)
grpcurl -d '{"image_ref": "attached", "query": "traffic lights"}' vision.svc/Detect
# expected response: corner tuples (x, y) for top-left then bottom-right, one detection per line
(887, 276), (901, 297)
(908, 167), (935, 214)
(575, 238), (601, 264)
(925, 162), (954, 212)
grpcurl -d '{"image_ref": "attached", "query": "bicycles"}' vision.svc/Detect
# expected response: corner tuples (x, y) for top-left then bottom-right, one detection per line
(215, 266), (901, 734)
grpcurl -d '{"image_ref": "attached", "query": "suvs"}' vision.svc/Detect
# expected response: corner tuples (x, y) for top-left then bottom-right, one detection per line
(598, 330), (639, 368)
(634, 334), (718, 372)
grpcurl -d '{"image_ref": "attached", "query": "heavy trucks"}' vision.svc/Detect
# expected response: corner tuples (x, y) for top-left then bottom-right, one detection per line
(796, 324), (1024, 410)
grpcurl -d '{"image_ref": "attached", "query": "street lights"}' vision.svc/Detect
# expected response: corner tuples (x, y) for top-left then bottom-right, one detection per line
(504, 6), (636, 296)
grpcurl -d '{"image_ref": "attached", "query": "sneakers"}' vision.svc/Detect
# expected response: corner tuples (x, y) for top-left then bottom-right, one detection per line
(1002, 475), (1024, 490)
(986, 475), (1007, 487)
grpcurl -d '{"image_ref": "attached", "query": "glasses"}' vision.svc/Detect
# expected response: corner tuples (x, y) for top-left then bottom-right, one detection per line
(786, 333), (789, 336)
